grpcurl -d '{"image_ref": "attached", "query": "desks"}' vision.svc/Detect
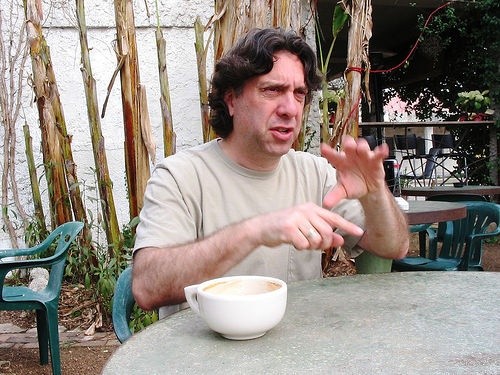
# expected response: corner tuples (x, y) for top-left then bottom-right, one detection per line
(358, 121), (496, 184)
(101, 271), (500, 375)
(399, 188), (500, 200)
(356, 201), (467, 274)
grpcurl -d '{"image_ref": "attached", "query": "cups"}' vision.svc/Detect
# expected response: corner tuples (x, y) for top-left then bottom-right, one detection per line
(183, 275), (287, 340)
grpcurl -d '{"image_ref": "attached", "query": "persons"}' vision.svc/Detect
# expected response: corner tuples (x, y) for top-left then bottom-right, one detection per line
(131, 26), (411, 321)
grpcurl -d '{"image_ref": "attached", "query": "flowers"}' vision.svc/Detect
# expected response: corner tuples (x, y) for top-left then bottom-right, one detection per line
(457, 89), (491, 112)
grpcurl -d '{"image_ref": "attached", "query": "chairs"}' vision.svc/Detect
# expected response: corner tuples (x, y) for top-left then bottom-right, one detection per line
(0, 222), (85, 375)
(393, 200), (500, 270)
(394, 133), (469, 189)
